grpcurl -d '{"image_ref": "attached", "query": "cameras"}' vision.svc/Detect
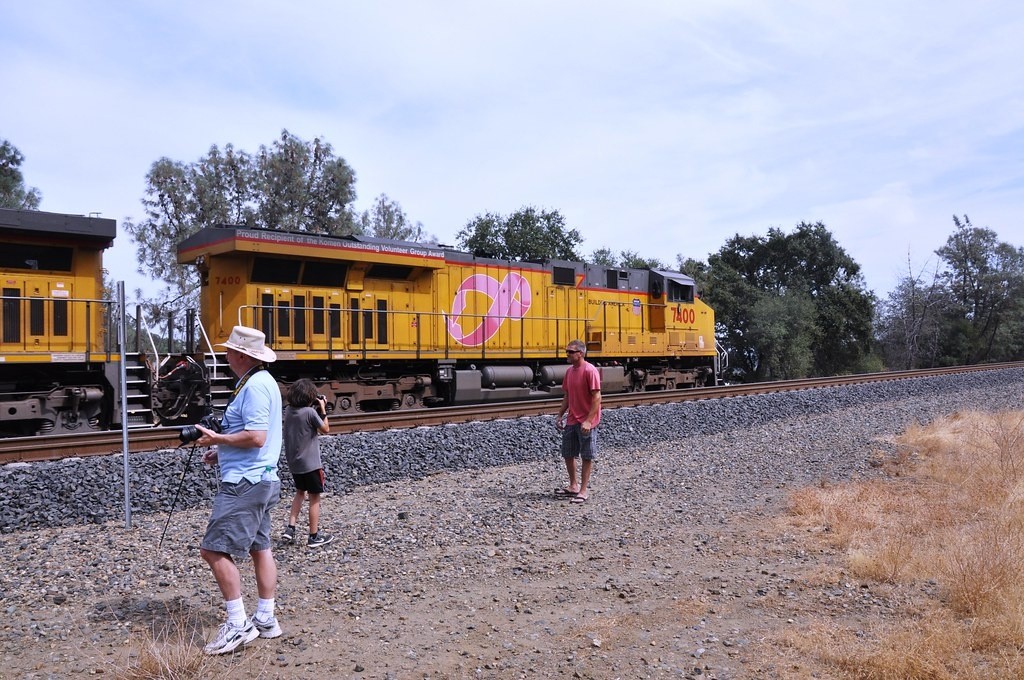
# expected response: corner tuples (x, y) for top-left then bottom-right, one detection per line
(178, 414), (222, 449)
(312, 395), (324, 406)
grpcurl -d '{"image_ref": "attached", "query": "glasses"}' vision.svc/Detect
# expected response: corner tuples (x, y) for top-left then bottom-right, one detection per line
(566, 350), (580, 354)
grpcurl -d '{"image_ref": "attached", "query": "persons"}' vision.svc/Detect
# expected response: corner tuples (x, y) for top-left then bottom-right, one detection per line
(555, 340), (601, 503)
(282, 378), (334, 548)
(195, 325), (283, 655)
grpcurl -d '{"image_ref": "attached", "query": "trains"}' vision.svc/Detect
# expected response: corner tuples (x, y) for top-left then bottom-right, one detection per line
(0, 207), (729, 438)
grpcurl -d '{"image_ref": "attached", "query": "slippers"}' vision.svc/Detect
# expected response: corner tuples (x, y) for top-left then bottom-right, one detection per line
(570, 497), (588, 503)
(555, 489), (579, 496)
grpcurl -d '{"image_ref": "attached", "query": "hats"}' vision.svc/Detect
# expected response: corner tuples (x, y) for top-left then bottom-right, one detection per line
(214, 325), (277, 363)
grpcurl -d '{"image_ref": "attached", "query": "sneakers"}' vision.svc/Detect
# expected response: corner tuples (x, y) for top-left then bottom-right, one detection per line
(306, 532), (334, 547)
(282, 527), (296, 539)
(202, 617), (259, 655)
(251, 614), (282, 638)
